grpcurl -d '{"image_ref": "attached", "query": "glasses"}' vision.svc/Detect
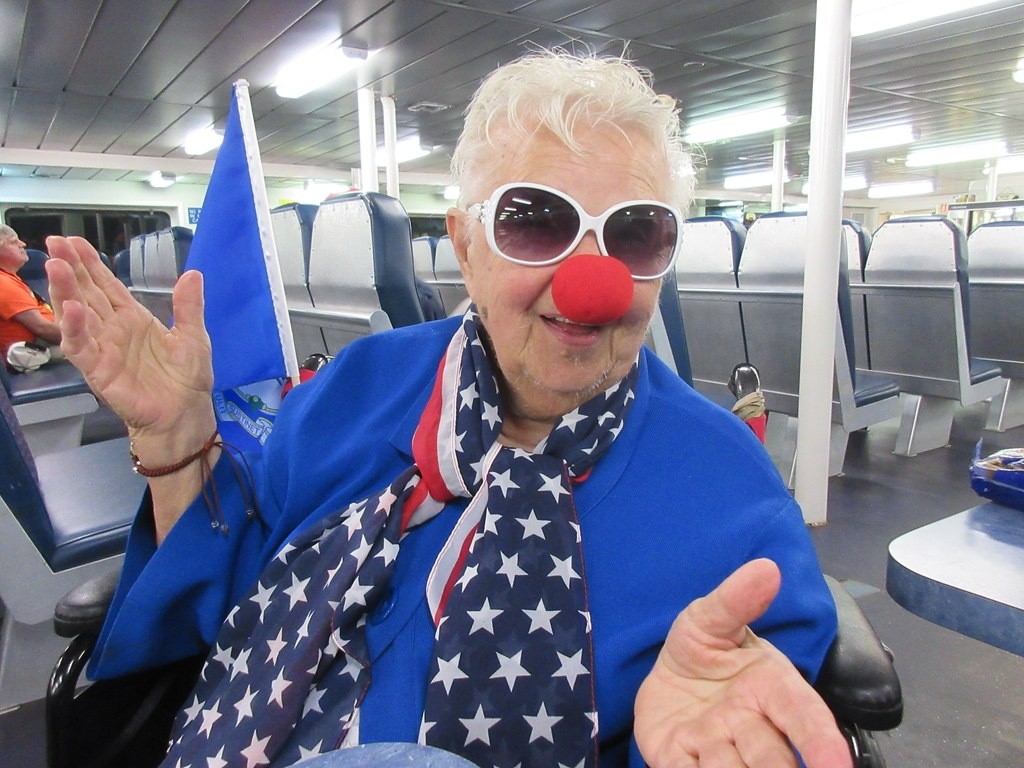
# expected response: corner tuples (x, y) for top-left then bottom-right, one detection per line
(468, 182), (681, 279)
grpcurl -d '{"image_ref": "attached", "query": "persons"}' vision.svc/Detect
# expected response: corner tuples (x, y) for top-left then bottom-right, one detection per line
(1, 224), (71, 374)
(43, 45), (852, 768)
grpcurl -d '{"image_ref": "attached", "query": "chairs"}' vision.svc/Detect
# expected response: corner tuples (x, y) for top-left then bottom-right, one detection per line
(0, 191), (1024, 626)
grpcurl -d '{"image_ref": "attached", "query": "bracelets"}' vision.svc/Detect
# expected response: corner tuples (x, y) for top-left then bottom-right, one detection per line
(129, 430), (258, 535)
